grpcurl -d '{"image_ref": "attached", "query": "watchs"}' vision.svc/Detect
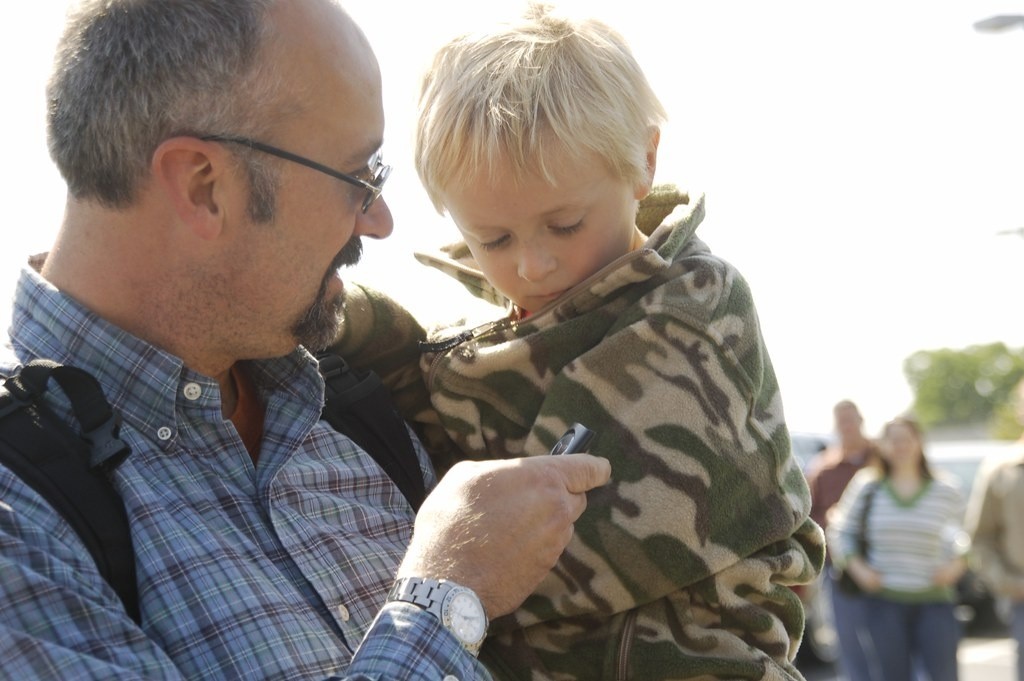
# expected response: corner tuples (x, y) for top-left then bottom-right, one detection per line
(387, 577), (489, 656)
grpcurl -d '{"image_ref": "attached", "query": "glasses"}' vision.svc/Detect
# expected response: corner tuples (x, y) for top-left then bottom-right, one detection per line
(181, 134), (388, 212)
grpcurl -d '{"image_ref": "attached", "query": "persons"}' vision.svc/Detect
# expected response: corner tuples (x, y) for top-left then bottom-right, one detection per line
(827, 416), (971, 681)
(0, 1), (612, 681)
(313, 0), (827, 681)
(968, 380), (1024, 681)
(806, 400), (872, 681)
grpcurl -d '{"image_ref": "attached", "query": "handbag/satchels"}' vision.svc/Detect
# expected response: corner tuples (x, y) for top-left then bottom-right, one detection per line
(841, 484), (877, 597)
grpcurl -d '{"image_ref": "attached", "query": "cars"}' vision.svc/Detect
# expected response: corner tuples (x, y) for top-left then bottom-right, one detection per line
(790, 435), (843, 666)
(922, 442), (1024, 622)
